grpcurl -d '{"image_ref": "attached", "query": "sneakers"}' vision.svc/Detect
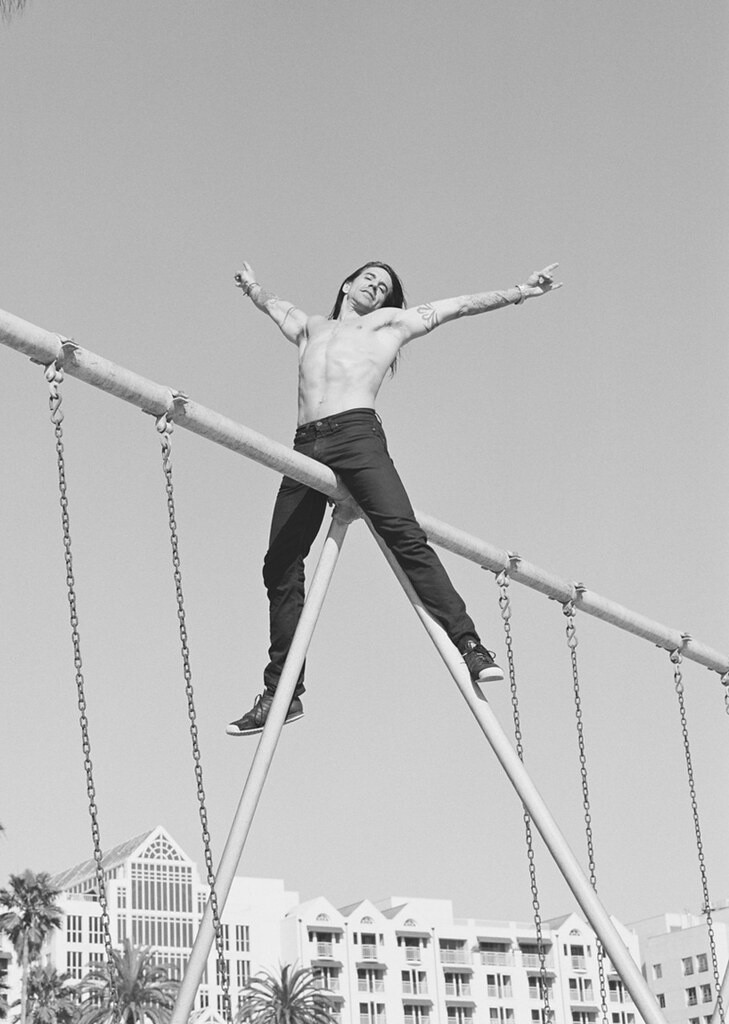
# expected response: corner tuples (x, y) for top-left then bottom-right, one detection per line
(226, 689), (306, 737)
(463, 643), (504, 682)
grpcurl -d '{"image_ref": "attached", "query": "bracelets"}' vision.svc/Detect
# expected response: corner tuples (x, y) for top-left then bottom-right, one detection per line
(243, 283), (258, 296)
(515, 284), (524, 305)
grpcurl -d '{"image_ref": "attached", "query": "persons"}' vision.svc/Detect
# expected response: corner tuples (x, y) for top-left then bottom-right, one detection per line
(225, 261), (564, 736)
